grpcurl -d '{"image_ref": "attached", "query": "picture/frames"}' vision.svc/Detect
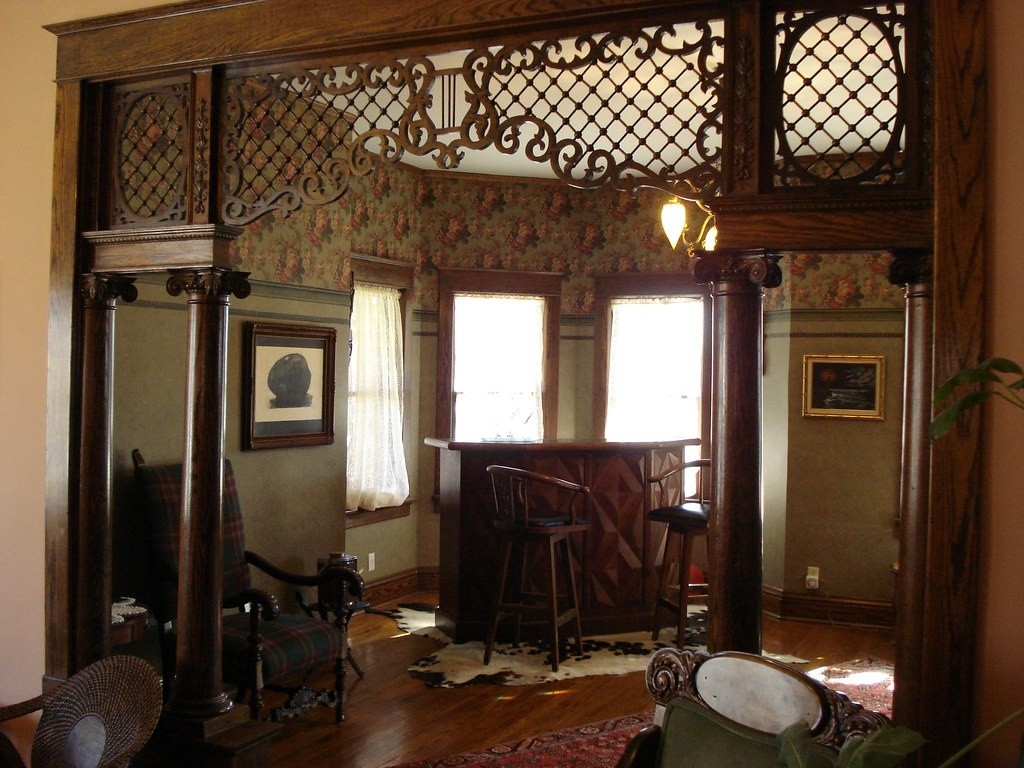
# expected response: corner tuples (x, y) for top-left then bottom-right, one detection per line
(241, 322), (337, 449)
(801, 352), (886, 420)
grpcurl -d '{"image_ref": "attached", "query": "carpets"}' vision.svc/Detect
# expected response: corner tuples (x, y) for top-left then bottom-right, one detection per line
(387, 658), (894, 768)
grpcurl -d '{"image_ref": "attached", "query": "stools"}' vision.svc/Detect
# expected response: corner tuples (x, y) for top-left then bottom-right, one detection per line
(646, 458), (711, 652)
(483, 463), (591, 673)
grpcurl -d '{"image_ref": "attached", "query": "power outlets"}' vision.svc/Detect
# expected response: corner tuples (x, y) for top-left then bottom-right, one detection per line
(805, 567), (819, 589)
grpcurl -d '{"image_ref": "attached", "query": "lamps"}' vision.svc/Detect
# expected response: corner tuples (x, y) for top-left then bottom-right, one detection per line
(661, 156), (720, 251)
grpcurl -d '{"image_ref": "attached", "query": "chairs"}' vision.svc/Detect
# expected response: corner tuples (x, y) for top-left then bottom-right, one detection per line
(0, 654), (163, 768)
(132, 447), (365, 724)
(616, 645), (931, 768)
